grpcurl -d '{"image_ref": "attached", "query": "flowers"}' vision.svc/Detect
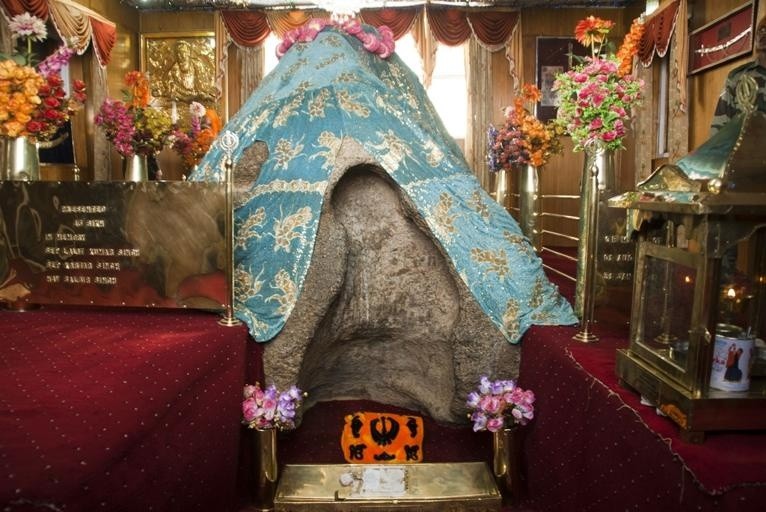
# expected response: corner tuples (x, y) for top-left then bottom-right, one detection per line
(240, 381), (309, 434)
(0, 11), (223, 169)
(484, 14), (651, 174)
(465, 377), (537, 435)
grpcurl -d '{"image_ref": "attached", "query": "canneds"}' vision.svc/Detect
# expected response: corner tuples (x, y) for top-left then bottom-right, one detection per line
(709, 322), (756, 392)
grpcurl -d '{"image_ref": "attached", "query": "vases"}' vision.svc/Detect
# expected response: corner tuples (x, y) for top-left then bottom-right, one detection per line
(496, 147), (616, 318)
(5, 135), (41, 183)
(493, 428), (517, 506)
(123, 153), (149, 181)
(253, 426), (278, 511)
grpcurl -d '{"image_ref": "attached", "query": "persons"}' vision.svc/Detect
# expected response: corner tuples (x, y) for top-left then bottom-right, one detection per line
(724, 348), (744, 381)
(122, 153), (162, 180)
